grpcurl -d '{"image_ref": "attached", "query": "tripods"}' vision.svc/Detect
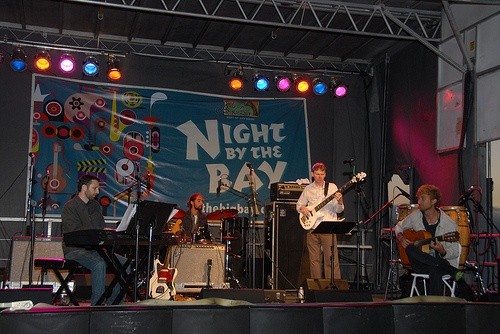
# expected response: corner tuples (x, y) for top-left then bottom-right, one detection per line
(310, 221), (356, 289)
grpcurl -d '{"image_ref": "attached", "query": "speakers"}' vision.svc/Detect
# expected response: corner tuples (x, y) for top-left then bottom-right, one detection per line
(264, 202), (311, 291)
(9, 236), (75, 293)
(168, 244), (226, 291)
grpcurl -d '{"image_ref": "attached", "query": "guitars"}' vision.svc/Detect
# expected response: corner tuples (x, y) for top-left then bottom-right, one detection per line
(398, 230), (461, 264)
(298, 171), (367, 231)
(147, 219), (182, 301)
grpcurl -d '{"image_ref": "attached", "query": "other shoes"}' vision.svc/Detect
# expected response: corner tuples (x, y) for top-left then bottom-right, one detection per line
(99, 303), (107, 306)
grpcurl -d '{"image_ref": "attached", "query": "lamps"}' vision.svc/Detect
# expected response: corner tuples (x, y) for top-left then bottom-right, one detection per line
(33, 49), (51, 71)
(275, 74), (290, 93)
(255, 71), (270, 93)
(331, 77), (346, 97)
(58, 49), (78, 75)
(82, 52), (101, 78)
(226, 65), (246, 91)
(107, 54), (124, 81)
(312, 74), (329, 96)
(10, 46), (28, 73)
(293, 73), (309, 93)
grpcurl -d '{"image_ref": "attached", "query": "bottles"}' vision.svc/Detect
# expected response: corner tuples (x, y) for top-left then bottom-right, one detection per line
(298, 287), (304, 304)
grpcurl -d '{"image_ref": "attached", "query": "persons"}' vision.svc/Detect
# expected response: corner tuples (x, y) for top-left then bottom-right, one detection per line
(61, 175), (130, 306)
(396, 182), (465, 295)
(297, 163), (344, 279)
(176, 193), (211, 241)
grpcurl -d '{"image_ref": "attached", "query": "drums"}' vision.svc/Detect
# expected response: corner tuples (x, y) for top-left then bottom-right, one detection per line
(396, 203), (470, 270)
(162, 218), (184, 236)
(166, 238), (222, 246)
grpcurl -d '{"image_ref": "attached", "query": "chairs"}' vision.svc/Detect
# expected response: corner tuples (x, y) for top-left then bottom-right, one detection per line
(407, 244), (463, 299)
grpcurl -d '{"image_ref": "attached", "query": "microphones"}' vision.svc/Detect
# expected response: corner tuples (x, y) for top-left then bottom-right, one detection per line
(457, 189), (474, 206)
(397, 186), (412, 200)
(343, 159), (354, 164)
(207, 259), (212, 270)
(461, 185), (474, 198)
(147, 172), (150, 190)
(217, 176), (221, 193)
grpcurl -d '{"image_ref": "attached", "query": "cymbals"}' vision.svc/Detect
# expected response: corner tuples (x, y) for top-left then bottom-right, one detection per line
(206, 208), (240, 221)
(171, 208), (186, 219)
(215, 236), (238, 240)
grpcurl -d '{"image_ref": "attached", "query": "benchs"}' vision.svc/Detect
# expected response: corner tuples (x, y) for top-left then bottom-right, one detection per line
(31, 256), (116, 306)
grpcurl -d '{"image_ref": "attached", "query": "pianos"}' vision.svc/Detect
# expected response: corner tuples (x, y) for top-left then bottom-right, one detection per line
(63, 228), (178, 306)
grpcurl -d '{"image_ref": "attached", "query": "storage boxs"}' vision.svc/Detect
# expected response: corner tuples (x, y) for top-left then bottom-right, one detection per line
(263, 201), (306, 292)
